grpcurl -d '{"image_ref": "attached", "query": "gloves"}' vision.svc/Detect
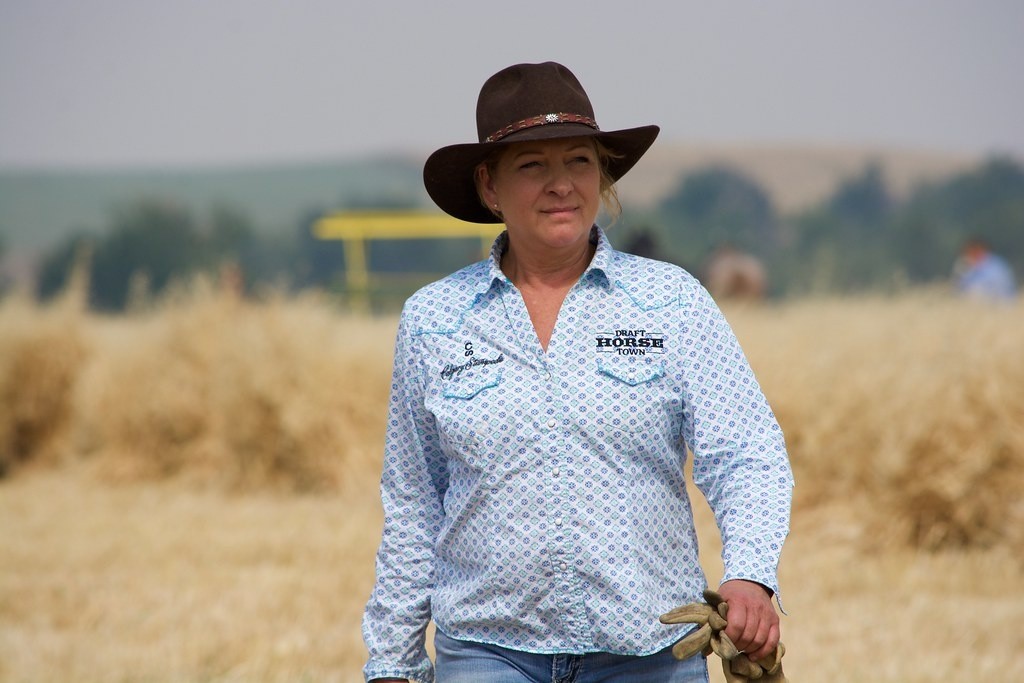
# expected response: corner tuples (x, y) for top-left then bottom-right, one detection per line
(659, 589), (789, 682)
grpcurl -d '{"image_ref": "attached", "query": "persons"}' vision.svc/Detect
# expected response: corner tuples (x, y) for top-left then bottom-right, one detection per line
(357, 64), (794, 682)
(950, 234), (1015, 301)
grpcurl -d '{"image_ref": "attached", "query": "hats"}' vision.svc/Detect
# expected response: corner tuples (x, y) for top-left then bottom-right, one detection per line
(423, 61), (660, 225)
